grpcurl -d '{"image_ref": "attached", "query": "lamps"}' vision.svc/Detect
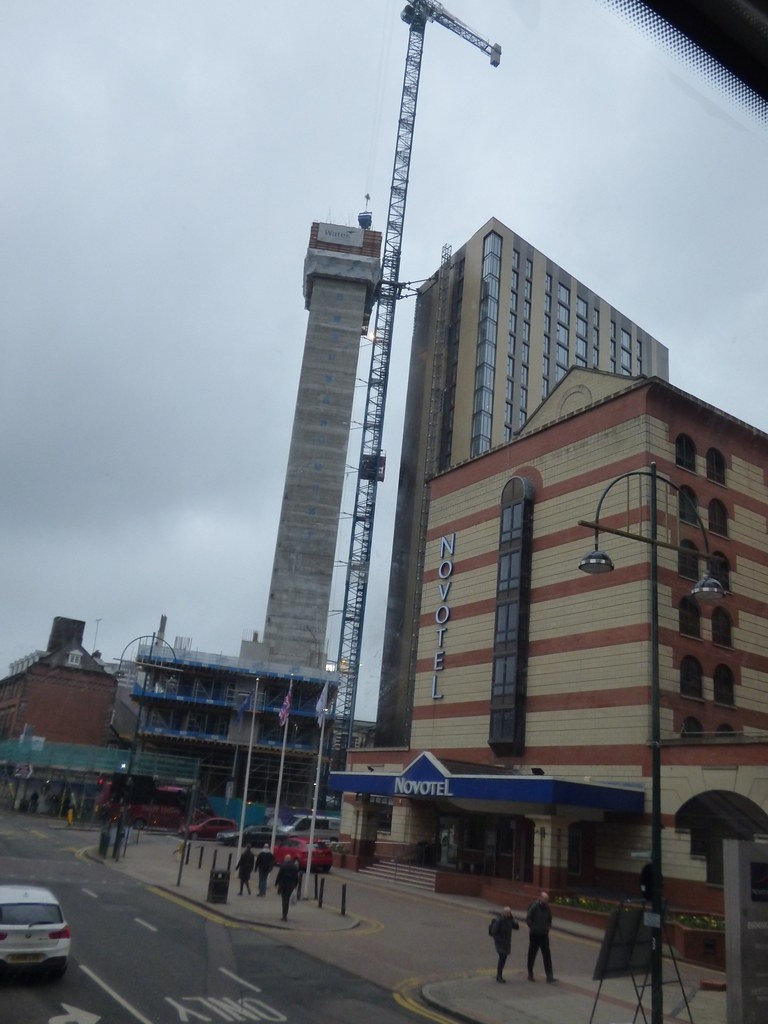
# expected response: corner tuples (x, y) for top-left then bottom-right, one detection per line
(530, 767), (544, 776)
(367, 766), (375, 773)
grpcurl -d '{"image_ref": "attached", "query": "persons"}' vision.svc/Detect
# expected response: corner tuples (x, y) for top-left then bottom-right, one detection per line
(255, 843), (274, 897)
(120, 824), (125, 842)
(50, 793), (71, 817)
(29, 790), (39, 813)
(236, 844), (254, 895)
(275, 854), (299, 922)
(526, 892), (559, 985)
(491, 906), (520, 983)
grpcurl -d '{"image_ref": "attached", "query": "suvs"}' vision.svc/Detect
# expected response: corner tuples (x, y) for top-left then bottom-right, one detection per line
(178, 817), (239, 840)
(0, 885), (71, 978)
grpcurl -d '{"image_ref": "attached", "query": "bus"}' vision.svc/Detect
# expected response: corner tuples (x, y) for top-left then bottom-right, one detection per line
(96, 772), (220, 829)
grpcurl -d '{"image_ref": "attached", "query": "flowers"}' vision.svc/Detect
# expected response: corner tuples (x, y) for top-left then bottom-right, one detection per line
(553, 896), (634, 913)
(329, 844), (348, 853)
(675, 914), (727, 932)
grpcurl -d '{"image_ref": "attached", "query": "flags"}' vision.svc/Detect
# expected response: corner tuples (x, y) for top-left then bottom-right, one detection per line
(278, 681), (293, 726)
(233, 690), (252, 727)
(316, 681), (328, 727)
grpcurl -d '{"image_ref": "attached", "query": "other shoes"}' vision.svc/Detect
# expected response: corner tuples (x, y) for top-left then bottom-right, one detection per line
(248, 891), (252, 895)
(496, 976), (505, 983)
(547, 977), (559, 984)
(282, 918), (287, 921)
(528, 976), (536, 982)
(238, 892), (243, 895)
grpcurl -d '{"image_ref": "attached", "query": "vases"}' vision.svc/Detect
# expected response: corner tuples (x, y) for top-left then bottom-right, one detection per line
(331, 851), (345, 868)
(674, 923), (730, 970)
(548, 905), (612, 929)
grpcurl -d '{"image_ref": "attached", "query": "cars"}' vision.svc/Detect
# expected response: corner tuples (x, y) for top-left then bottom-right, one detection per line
(216, 825), (290, 847)
(270, 836), (333, 873)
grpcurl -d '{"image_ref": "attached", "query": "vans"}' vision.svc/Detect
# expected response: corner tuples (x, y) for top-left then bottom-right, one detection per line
(278, 814), (341, 841)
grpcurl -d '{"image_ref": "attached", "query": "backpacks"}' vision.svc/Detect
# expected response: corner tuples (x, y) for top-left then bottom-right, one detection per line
(488, 918), (500, 936)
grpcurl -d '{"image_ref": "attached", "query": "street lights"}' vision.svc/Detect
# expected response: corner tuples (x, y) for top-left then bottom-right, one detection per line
(98, 632), (184, 864)
(578, 459), (728, 1024)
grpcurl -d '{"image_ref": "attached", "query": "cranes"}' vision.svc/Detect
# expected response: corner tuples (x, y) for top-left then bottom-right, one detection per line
(318, 0), (501, 813)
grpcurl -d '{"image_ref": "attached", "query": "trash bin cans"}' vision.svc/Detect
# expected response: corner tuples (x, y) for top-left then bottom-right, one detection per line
(417, 841), (432, 866)
(19, 799), (30, 812)
(207, 869), (231, 904)
